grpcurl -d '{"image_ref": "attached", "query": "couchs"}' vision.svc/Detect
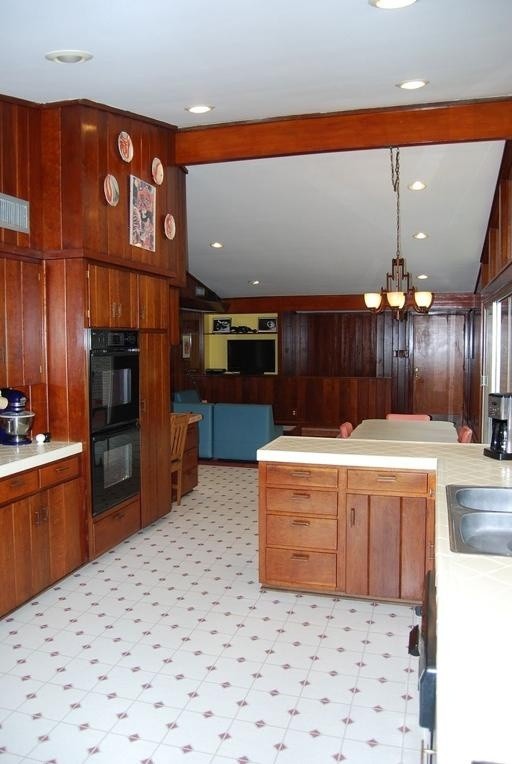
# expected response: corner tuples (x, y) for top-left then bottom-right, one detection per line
(213, 403), (284, 462)
(172, 402), (211, 461)
(172, 389), (200, 404)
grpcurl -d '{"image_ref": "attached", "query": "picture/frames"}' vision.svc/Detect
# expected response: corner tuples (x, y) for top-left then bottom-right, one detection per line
(181, 334), (192, 358)
(211, 317), (232, 334)
(128, 173), (158, 253)
(257, 316), (279, 333)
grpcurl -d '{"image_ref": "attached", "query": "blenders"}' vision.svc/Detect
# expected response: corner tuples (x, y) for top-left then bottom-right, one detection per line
(0, 388), (35, 445)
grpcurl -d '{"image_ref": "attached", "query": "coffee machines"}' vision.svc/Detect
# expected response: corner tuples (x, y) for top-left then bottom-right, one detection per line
(483, 392), (512, 461)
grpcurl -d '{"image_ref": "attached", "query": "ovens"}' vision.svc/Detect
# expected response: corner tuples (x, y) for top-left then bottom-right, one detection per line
(87, 327), (140, 434)
(89, 426), (142, 516)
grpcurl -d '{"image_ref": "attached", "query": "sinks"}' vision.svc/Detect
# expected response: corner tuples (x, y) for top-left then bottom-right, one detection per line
(446, 484), (512, 511)
(448, 511), (512, 557)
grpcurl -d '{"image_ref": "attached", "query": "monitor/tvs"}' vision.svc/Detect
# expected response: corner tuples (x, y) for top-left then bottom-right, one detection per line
(227, 339), (276, 375)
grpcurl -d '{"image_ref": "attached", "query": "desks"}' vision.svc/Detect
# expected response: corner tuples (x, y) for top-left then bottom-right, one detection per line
(172, 412), (203, 502)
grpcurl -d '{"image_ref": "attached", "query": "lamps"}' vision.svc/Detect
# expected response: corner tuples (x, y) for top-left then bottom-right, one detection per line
(362, 147), (434, 322)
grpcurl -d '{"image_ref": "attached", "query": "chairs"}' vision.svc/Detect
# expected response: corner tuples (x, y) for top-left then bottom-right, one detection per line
(169, 411), (192, 506)
(386, 414), (430, 423)
(339, 421), (353, 438)
(458, 424), (473, 444)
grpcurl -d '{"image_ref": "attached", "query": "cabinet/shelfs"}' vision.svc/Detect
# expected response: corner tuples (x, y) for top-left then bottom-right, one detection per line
(259, 461), (437, 602)
(0, 450), (83, 623)
(87, 264), (170, 331)
